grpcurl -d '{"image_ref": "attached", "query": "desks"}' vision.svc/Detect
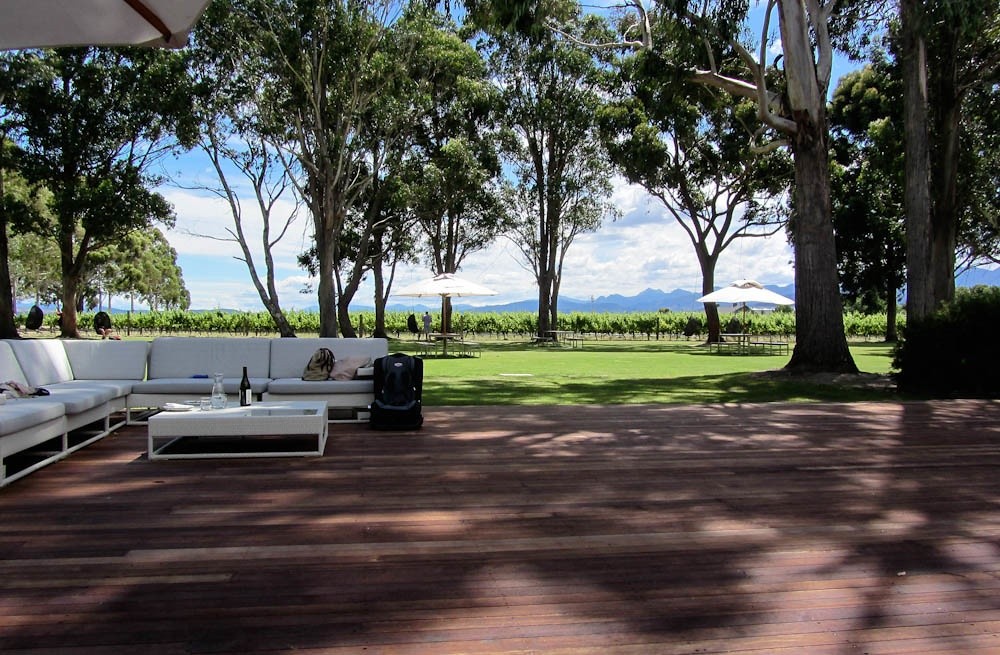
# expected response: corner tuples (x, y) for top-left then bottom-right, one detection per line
(427, 332), (464, 358)
(718, 333), (752, 355)
(543, 329), (575, 348)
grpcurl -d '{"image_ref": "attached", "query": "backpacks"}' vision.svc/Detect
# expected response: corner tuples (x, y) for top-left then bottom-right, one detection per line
(370, 353), (424, 430)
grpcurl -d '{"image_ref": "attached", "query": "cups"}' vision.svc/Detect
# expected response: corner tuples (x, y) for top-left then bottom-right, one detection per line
(200, 397), (211, 411)
(213, 396), (227, 408)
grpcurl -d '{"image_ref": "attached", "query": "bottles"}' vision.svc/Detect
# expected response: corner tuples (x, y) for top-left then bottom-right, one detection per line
(212, 372), (224, 407)
(238, 366), (252, 406)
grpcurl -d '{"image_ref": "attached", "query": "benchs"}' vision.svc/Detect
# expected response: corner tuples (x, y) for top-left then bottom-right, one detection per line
(533, 336), (583, 348)
(127, 337), (388, 423)
(710, 342), (740, 354)
(750, 342), (789, 355)
(416, 340), (481, 358)
(0, 339), (149, 488)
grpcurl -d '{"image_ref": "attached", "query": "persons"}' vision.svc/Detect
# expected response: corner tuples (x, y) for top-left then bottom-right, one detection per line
(97, 314), (112, 339)
(56, 307), (63, 331)
(422, 311), (432, 339)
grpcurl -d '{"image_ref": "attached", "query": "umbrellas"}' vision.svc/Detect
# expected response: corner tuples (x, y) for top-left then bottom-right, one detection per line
(696, 278), (796, 354)
(0, 0), (213, 51)
(390, 273), (497, 337)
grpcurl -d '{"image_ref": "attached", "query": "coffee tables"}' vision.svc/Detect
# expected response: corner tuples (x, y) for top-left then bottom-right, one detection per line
(148, 399), (328, 458)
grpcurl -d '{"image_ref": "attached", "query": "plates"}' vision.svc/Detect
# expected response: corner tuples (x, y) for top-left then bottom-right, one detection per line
(184, 401), (211, 405)
(163, 405), (195, 410)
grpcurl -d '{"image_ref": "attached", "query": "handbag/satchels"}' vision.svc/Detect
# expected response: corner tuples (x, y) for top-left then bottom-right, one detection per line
(301, 347), (335, 381)
(329, 354), (372, 381)
